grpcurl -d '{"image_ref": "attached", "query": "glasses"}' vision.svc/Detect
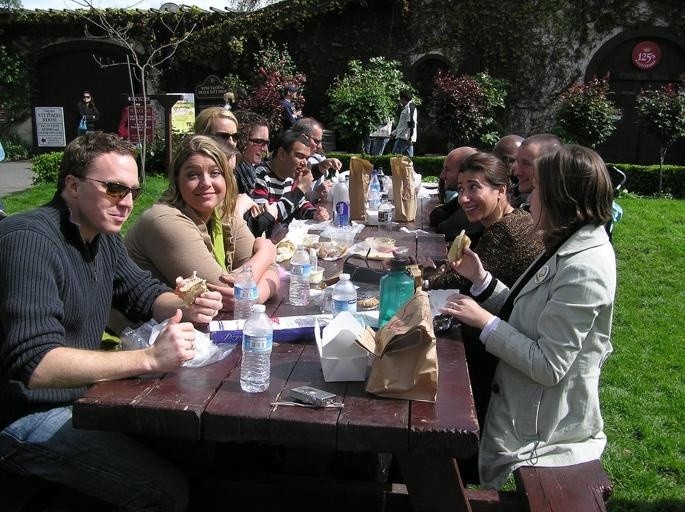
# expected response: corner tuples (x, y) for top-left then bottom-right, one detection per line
(211, 132), (239, 141)
(306, 135), (322, 144)
(84, 96), (89, 98)
(83, 177), (142, 200)
(249, 139), (270, 144)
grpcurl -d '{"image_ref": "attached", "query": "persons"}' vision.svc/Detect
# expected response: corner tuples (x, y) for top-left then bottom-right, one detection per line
(0, 130), (224, 512)
(100, 134), (280, 350)
(118, 92), (130, 139)
(436, 142), (618, 492)
(354, 89), (419, 157)
(380, 134), (563, 344)
(74, 89), (99, 139)
(195, 82), (343, 242)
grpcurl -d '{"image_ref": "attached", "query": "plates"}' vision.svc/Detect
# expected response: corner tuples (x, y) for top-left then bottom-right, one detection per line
(360, 306), (378, 311)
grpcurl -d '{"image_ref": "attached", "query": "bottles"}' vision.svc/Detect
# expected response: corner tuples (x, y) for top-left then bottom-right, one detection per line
(310, 249), (317, 271)
(368, 170), (380, 209)
(377, 194), (393, 236)
(330, 274), (356, 316)
(240, 304), (273, 393)
(378, 258), (414, 330)
(332, 176), (350, 223)
(287, 244), (311, 307)
(234, 266), (257, 322)
(378, 167), (384, 191)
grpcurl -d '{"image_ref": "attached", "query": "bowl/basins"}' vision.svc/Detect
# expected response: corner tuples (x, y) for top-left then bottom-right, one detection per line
(311, 266), (325, 282)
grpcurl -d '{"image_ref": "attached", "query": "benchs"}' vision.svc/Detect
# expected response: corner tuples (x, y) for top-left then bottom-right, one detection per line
(518, 459), (614, 512)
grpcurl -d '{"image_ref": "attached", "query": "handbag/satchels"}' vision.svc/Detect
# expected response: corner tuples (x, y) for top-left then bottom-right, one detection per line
(79, 115), (87, 130)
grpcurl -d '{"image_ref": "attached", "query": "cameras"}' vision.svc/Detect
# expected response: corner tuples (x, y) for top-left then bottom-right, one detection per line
(290, 385), (345, 408)
